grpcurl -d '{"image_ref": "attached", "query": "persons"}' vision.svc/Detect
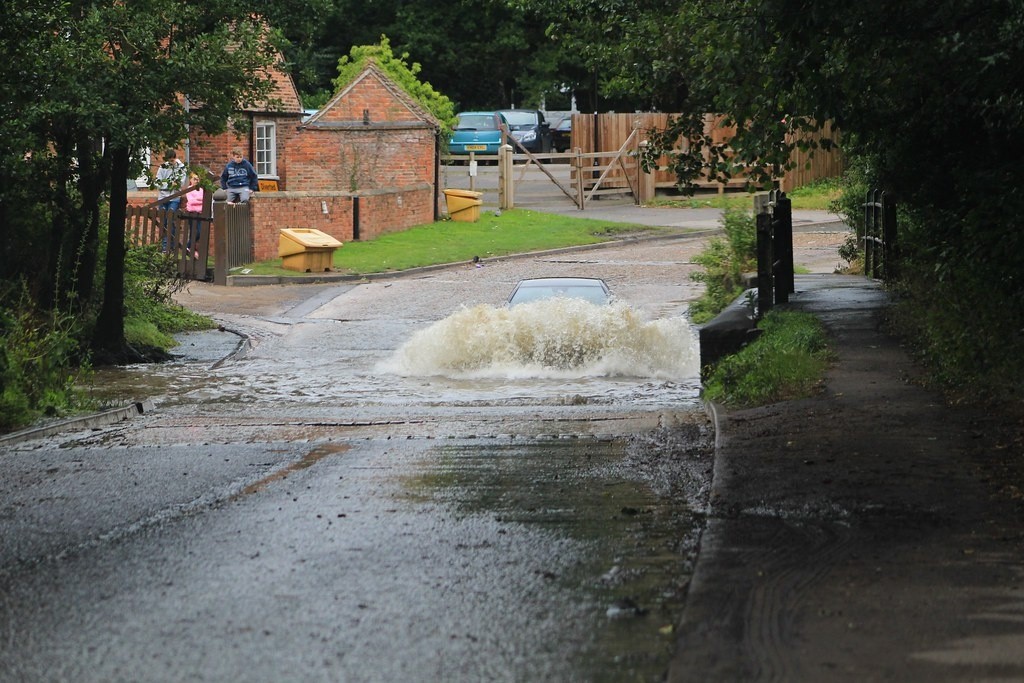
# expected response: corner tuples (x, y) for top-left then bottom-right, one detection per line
(153, 149), (187, 253)
(185, 173), (203, 260)
(221, 147), (258, 204)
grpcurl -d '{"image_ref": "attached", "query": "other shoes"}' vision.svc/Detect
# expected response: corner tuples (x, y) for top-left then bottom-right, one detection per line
(185, 247), (199, 260)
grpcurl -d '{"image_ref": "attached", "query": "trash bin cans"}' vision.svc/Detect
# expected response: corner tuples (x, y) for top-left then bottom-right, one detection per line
(442, 189), (483, 222)
(278, 228), (344, 273)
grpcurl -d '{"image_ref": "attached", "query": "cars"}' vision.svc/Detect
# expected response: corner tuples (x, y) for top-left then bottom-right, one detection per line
(448, 111), (516, 164)
(496, 109), (553, 164)
(502, 278), (615, 310)
(544, 111), (580, 153)
